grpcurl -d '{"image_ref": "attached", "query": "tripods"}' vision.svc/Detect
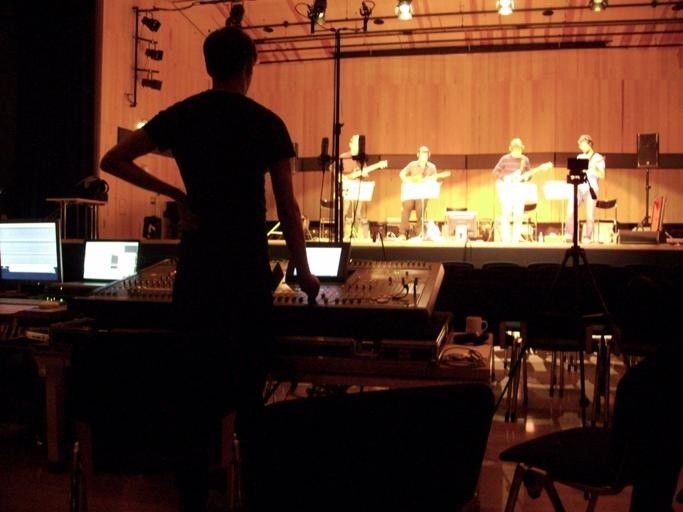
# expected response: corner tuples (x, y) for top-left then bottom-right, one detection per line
(632, 168), (675, 240)
(491, 178), (629, 426)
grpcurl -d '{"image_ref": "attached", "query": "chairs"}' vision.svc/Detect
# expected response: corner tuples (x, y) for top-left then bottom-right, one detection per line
(518, 203), (538, 243)
(593, 198), (617, 245)
(498, 358), (657, 512)
(319, 199), (336, 239)
(47, 320), (496, 511)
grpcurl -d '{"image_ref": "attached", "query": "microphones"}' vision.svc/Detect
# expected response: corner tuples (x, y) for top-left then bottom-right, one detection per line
(359, 135), (365, 170)
(321, 137), (328, 174)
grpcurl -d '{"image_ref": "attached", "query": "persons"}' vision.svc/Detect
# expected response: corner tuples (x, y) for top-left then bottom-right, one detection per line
(100, 28), (320, 512)
(329, 134), (443, 242)
(490, 134), (605, 247)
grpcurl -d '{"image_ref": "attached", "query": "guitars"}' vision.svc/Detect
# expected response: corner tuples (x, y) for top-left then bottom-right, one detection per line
(401, 170), (451, 183)
(496, 161), (552, 188)
(338, 160), (388, 183)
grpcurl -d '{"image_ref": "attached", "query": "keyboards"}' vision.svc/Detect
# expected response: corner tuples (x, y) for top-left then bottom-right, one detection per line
(0, 297), (42, 305)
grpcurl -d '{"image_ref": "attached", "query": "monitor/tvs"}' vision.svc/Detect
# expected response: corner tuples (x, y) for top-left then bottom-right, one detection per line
(0, 219), (63, 296)
(285, 242), (350, 282)
(82, 239), (141, 281)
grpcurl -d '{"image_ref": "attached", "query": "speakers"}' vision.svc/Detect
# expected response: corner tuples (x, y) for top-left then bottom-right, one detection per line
(637, 133), (659, 168)
(620, 230), (666, 244)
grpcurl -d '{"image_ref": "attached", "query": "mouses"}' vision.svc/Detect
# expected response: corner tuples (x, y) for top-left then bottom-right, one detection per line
(40, 301), (59, 309)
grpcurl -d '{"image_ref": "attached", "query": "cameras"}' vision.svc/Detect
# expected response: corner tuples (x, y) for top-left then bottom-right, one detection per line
(568, 159), (588, 174)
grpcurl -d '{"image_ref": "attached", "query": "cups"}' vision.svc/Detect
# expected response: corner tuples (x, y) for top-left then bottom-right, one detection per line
(466, 317), (489, 336)
